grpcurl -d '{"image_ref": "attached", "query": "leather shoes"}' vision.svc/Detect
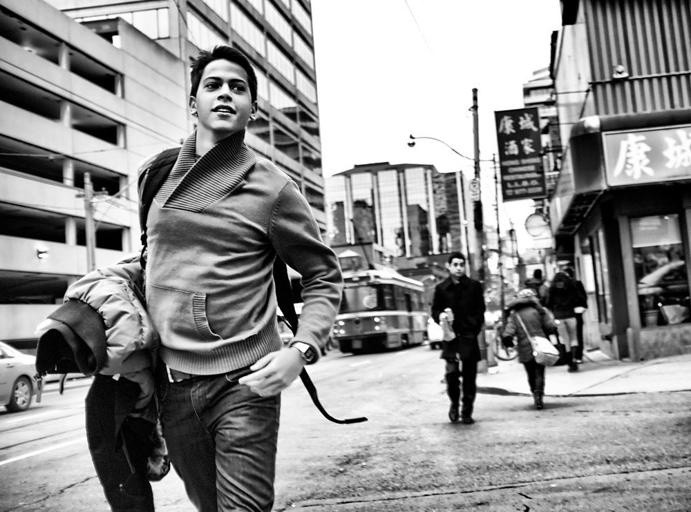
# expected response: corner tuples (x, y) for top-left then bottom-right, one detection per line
(463, 417), (473, 424)
(449, 406), (459, 422)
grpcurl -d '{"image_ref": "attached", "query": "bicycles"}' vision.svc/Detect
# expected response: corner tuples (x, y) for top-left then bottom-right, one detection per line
(491, 317), (520, 363)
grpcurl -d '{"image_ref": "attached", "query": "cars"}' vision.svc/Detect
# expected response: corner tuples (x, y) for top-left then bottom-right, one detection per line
(272, 318), (337, 359)
(638, 260), (688, 307)
(427, 310), (450, 351)
(0, 340), (45, 412)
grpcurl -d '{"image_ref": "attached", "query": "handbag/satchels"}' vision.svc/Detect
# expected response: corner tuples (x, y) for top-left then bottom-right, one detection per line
(531, 336), (560, 366)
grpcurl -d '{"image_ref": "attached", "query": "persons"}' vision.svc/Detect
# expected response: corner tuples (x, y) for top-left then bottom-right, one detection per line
(430, 250), (486, 423)
(135, 46), (343, 511)
(498, 287), (559, 411)
(526, 263), (588, 373)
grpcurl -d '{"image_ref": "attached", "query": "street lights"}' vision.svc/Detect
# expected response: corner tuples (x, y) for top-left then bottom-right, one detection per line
(407, 132), (508, 315)
(460, 218), (503, 243)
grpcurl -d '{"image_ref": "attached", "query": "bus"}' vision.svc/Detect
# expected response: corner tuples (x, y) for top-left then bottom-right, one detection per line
(332, 269), (428, 354)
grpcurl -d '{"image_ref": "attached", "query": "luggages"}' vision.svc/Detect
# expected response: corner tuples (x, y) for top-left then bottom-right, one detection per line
(546, 332), (566, 365)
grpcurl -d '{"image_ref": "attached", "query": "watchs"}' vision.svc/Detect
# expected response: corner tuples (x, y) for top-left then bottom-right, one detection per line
(290, 342), (318, 367)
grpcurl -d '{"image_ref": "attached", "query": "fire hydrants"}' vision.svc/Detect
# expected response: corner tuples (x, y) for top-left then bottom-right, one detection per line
(485, 331), (497, 368)
